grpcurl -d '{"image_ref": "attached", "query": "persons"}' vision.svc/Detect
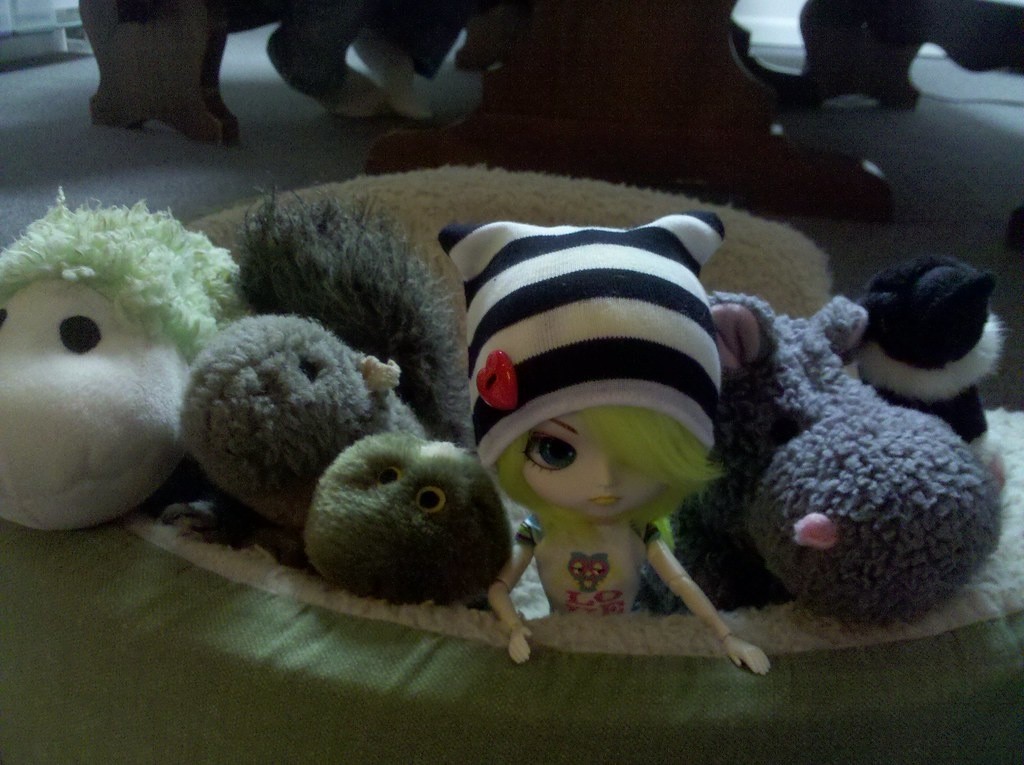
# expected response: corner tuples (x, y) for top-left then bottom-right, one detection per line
(266, 0), (469, 120)
(438, 210), (772, 676)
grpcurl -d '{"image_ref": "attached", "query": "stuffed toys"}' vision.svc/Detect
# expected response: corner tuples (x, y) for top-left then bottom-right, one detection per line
(0, 191), (513, 609)
(633, 258), (1007, 629)
